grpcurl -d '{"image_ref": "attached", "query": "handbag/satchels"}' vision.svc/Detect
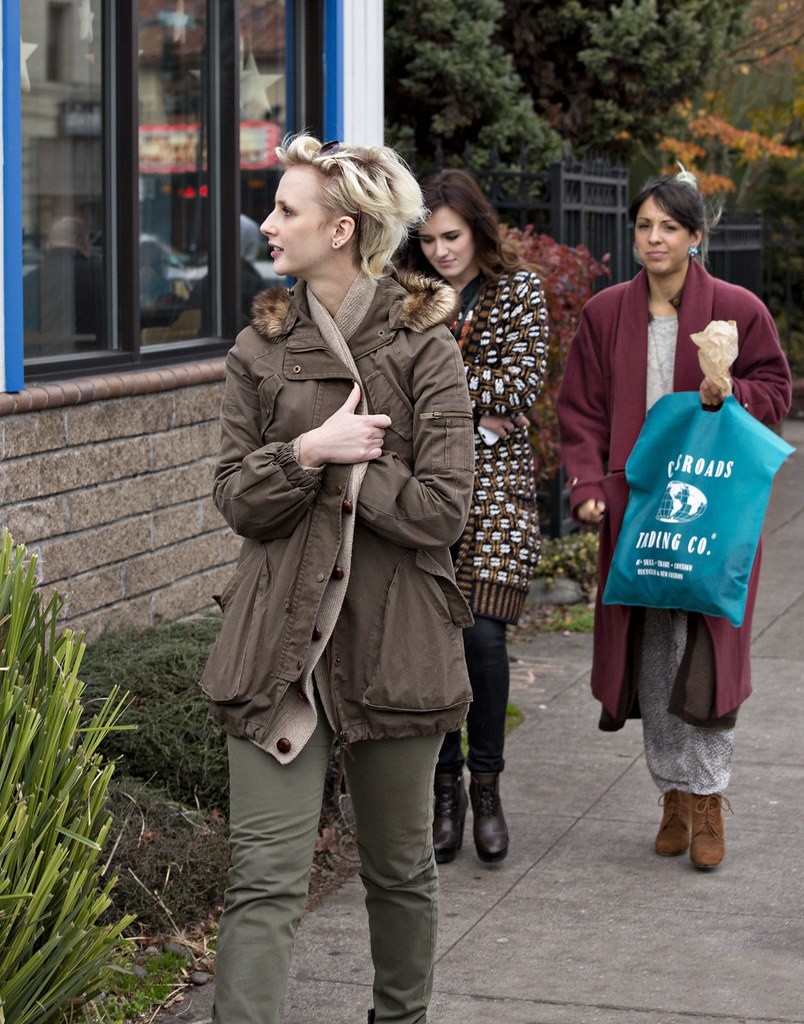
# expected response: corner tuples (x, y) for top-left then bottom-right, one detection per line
(601, 391), (799, 628)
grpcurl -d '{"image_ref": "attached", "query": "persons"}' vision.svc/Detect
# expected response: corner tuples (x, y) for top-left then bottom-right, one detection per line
(556, 179), (792, 870)
(399, 170), (549, 862)
(140, 213), (269, 340)
(199, 133), (476, 1024)
(23, 215), (109, 357)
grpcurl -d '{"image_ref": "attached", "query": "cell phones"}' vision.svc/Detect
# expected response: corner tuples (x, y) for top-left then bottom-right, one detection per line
(478, 426), (499, 446)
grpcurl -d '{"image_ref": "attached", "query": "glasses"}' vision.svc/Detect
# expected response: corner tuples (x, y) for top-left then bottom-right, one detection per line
(320, 141), (349, 194)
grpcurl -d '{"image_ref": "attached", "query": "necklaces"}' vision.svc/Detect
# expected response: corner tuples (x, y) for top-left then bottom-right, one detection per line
(645, 296), (676, 392)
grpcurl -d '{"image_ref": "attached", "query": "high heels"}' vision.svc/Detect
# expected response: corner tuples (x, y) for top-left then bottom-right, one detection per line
(430, 775), (469, 863)
(471, 771), (508, 864)
(655, 787), (695, 855)
(690, 791), (734, 870)
